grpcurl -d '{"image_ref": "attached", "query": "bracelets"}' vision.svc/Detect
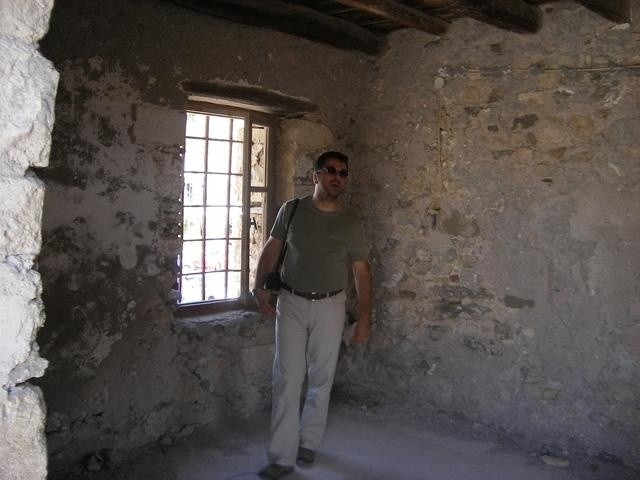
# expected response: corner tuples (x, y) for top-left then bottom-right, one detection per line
(250, 284), (264, 298)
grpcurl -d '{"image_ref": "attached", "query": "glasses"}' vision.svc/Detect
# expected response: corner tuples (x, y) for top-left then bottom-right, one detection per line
(314, 166), (349, 178)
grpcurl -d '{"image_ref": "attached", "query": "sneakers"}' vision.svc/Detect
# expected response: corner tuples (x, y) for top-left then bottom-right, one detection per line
(258, 464), (294, 478)
(296, 447), (315, 467)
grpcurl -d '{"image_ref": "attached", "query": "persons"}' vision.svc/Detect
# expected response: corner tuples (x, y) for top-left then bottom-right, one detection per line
(249, 149), (379, 478)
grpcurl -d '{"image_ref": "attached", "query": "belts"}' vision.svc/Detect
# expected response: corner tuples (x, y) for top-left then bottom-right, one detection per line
(280, 282), (344, 301)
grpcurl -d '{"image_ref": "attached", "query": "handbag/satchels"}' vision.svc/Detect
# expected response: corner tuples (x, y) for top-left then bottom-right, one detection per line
(261, 272), (281, 295)
